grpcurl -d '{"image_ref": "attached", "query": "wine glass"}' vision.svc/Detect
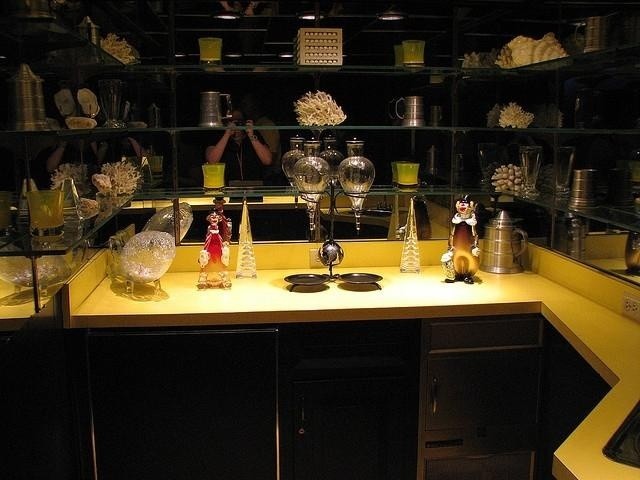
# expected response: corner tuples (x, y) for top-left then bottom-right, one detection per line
(518, 144), (544, 196)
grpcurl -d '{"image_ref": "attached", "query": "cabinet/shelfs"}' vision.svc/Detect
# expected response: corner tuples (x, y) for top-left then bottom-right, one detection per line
(0, 0), (640, 312)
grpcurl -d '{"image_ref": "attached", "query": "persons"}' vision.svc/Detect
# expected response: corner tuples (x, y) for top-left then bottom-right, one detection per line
(494, 134), (553, 167)
(201, 92), (273, 186)
(196, 210), (232, 292)
(440, 186), (481, 284)
(30, 105), (84, 189)
(87, 137), (152, 172)
(206, 192), (234, 270)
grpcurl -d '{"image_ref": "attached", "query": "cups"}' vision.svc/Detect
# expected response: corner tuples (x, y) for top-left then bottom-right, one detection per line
(394, 95), (427, 127)
(569, 166), (605, 209)
(573, 14), (615, 54)
(197, 90), (234, 128)
(607, 166), (637, 210)
(552, 146), (578, 200)
(430, 105), (444, 126)
(477, 140), (498, 187)
(97, 78), (131, 129)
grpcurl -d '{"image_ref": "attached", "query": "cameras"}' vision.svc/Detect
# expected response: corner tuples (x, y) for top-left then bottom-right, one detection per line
(236, 120), (246, 126)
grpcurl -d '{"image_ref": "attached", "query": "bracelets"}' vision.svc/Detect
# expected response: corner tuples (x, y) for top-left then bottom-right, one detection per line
(248, 133), (259, 141)
